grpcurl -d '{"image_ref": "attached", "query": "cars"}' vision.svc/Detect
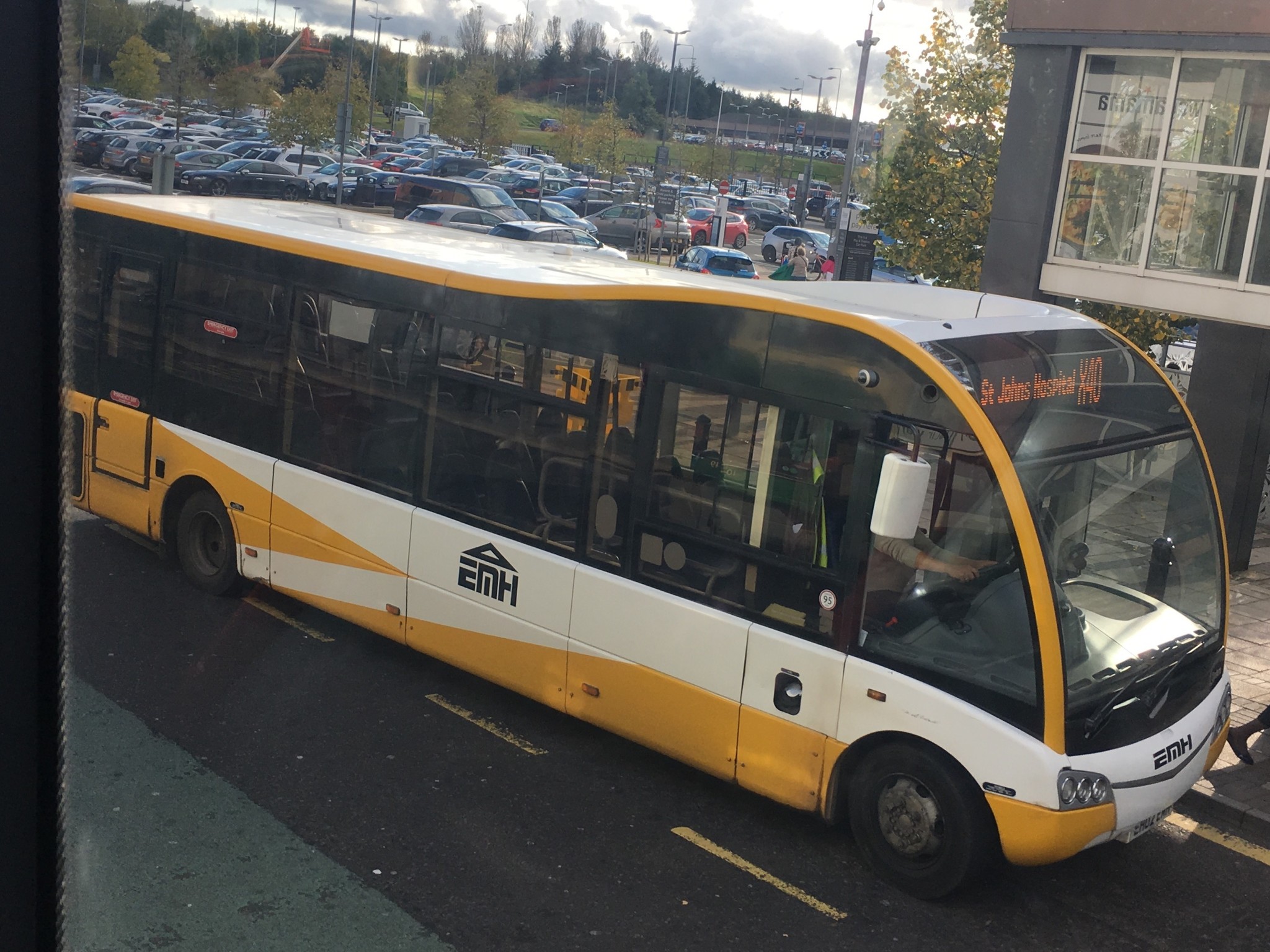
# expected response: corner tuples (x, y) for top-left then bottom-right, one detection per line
(403, 204), (506, 235)
(486, 221), (628, 261)
(761, 226), (834, 263)
(580, 202), (693, 255)
(683, 207), (749, 249)
(73, 82), (987, 251)
(676, 246), (760, 279)
(871, 256), (941, 288)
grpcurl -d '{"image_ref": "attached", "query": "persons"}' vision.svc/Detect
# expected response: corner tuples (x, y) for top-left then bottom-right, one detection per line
(788, 246), (809, 281)
(862, 523), (999, 624)
(788, 238), (802, 260)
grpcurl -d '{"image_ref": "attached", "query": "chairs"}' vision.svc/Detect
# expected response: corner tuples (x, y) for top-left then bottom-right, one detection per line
(78, 290), (755, 587)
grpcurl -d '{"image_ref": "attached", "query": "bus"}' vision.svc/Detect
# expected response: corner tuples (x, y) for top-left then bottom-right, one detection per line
(73, 178), (1233, 900)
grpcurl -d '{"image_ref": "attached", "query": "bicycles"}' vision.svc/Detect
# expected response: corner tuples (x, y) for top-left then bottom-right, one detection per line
(805, 247), (827, 281)
